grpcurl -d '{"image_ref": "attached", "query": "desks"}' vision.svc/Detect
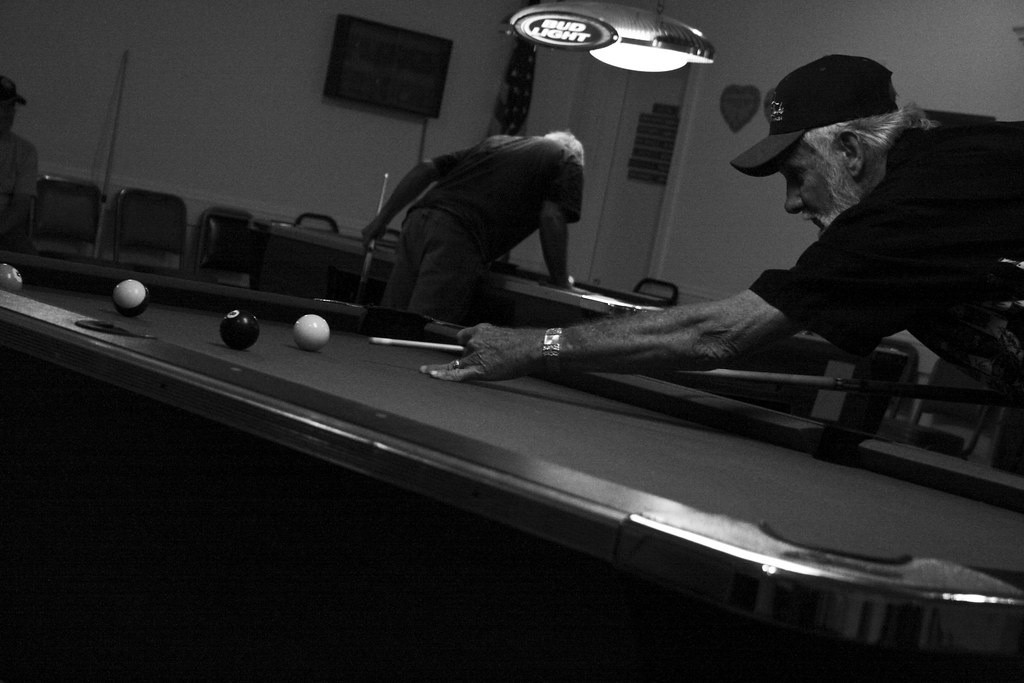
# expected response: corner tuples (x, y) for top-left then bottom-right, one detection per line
(1, 246), (1023, 683)
(252, 219), (920, 430)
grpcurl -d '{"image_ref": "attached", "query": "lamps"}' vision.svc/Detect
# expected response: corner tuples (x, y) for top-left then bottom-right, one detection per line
(543, 0), (715, 72)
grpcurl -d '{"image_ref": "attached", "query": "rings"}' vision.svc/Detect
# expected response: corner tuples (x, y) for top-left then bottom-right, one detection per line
(453, 359), (459, 369)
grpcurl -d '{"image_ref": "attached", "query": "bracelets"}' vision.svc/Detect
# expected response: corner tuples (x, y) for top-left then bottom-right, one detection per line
(542, 328), (563, 375)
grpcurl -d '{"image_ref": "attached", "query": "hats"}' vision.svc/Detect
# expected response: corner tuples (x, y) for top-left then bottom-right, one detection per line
(0, 76), (26, 106)
(730, 54), (897, 177)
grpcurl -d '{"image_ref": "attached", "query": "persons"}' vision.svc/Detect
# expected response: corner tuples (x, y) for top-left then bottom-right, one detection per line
(0, 75), (40, 284)
(362, 131), (586, 322)
(420, 51), (1024, 394)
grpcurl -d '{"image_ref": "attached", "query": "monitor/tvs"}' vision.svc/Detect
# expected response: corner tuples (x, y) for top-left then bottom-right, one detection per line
(323, 14), (454, 120)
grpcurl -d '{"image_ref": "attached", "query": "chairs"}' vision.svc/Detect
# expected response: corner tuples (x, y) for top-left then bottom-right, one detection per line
(876, 361), (994, 453)
(30, 176), (271, 288)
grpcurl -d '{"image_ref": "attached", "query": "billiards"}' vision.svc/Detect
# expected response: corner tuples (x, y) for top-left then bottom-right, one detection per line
(112, 277), (152, 318)
(292, 313), (331, 352)
(568, 275), (575, 286)
(219, 309), (262, 352)
(0, 262), (23, 293)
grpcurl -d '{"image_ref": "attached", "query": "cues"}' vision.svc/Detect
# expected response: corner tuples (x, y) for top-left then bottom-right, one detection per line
(367, 333), (1024, 410)
(92, 46), (130, 262)
(355, 172), (390, 303)
(411, 117), (430, 206)
